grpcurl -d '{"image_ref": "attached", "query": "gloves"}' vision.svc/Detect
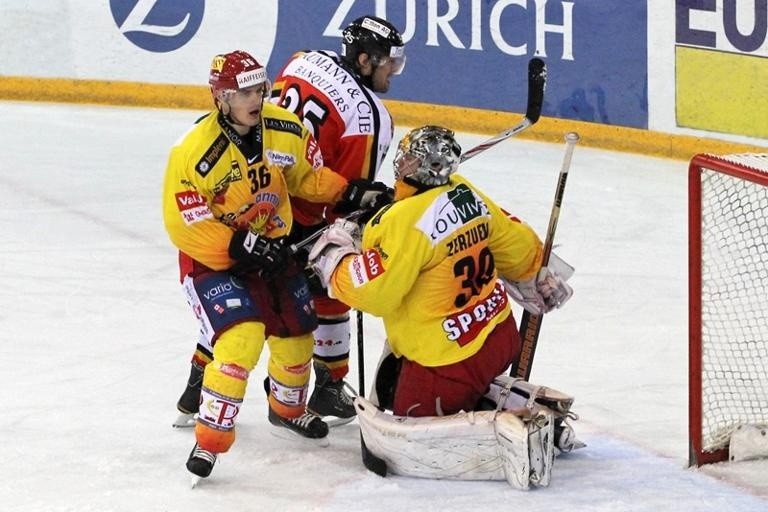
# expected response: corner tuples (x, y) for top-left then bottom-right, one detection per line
(228, 230), (292, 268)
(341, 178), (395, 222)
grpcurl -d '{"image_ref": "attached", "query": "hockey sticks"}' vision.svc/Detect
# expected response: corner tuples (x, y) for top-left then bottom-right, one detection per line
(290, 56), (547, 255)
(357, 311), (387, 477)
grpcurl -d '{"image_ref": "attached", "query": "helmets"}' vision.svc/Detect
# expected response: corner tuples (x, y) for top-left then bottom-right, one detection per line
(342, 15), (406, 76)
(208, 51), (272, 102)
(394, 126), (461, 185)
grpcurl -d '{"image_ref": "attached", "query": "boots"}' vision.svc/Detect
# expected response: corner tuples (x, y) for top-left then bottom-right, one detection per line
(187, 439), (218, 477)
(176, 358), (205, 416)
(263, 377), (329, 438)
(307, 362), (364, 419)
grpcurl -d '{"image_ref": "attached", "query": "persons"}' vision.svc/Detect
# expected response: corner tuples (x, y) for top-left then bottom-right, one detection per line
(161, 48), (393, 479)
(310, 126), (576, 491)
(178, 15), (405, 422)
